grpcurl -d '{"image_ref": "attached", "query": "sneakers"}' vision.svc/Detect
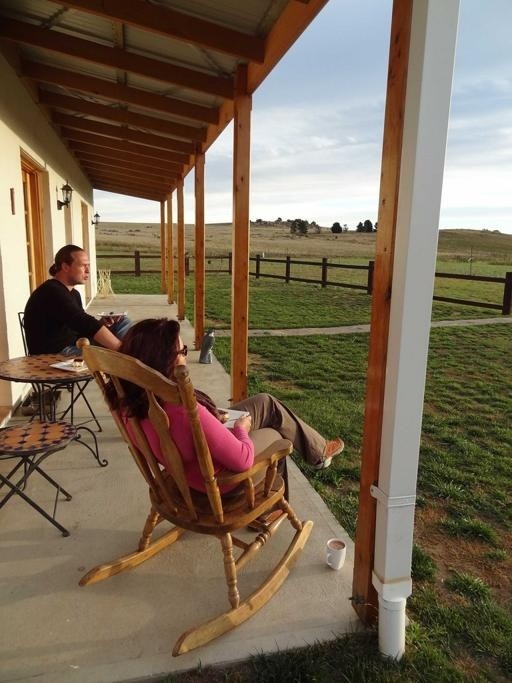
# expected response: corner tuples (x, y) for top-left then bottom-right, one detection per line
(313, 437), (344, 469)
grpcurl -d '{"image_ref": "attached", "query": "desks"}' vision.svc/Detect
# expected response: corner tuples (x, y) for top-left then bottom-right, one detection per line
(0, 422), (77, 537)
(0, 354), (108, 467)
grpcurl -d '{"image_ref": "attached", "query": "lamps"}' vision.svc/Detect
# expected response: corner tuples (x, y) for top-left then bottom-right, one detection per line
(57, 180), (73, 210)
(92, 212), (100, 224)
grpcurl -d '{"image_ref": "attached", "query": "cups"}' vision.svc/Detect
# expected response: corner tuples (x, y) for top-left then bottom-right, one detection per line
(325, 538), (347, 570)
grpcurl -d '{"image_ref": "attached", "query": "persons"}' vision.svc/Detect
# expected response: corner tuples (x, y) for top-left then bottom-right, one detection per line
(101, 317), (345, 533)
(24, 245), (133, 355)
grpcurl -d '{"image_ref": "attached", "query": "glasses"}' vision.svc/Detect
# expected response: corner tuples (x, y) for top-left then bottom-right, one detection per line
(178, 345), (187, 356)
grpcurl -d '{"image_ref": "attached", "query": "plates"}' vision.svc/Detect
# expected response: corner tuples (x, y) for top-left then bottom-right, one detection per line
(49, 358), (89, 373)
(96, 312), (127, 317)
(217, 408), (250, 429)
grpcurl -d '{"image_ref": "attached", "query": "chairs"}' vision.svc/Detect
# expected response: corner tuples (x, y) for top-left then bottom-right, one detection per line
(19, 312), (103, 433)
(76, 338), (314, 656)
